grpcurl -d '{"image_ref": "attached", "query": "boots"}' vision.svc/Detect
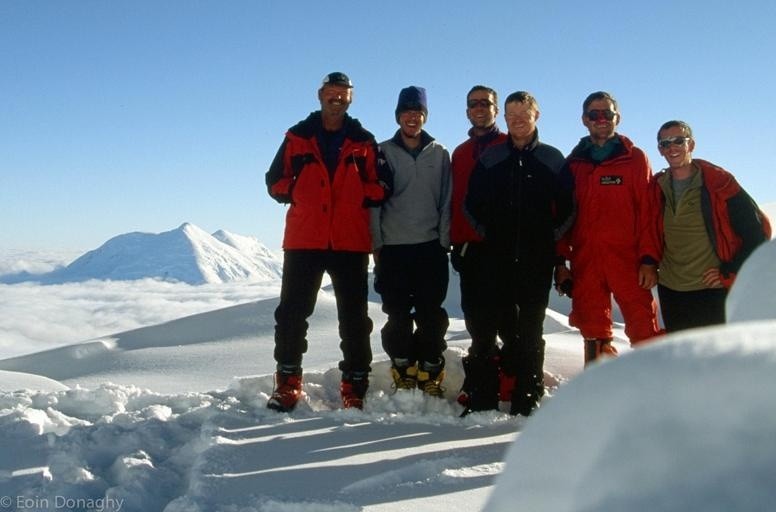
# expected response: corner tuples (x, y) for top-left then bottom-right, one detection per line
(266, 357), (545, 415)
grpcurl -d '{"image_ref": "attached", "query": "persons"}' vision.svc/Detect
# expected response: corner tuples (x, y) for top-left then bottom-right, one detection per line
(266, 70), (394, 413)
(449, 84), (510, 418)
(652, 117), (771, 334)
(372, 85), (454, 402)
(459, 90), (578, 418)
(554, 92), (667, 370)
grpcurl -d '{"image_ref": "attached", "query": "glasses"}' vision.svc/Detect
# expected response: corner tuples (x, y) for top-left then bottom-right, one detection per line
(468, 98), (497, 108)
(588, 109), (614, 122)
(658, 137), (691, 150)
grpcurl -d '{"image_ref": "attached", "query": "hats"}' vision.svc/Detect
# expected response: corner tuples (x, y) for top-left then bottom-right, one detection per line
(395, 86), (428, 124)
(323, 71), (353, 88)
